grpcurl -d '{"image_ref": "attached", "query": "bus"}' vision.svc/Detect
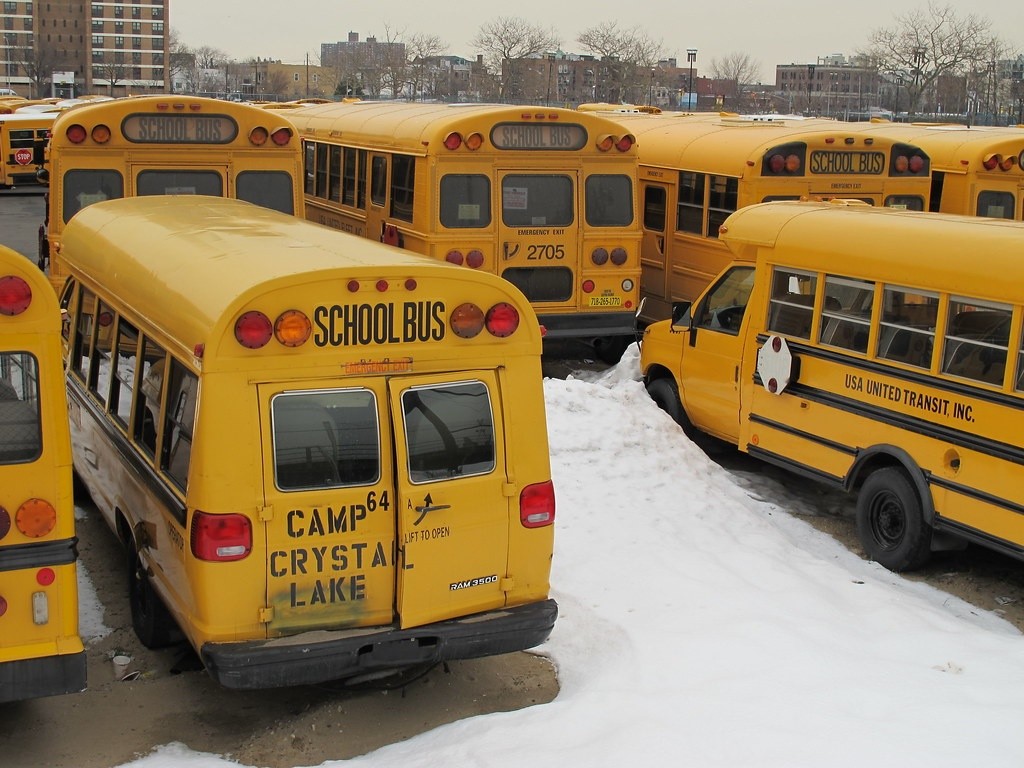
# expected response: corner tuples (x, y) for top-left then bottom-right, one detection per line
(575, 102), (933, 336)
(0, 89), (119, 189)
(37, 94), (306, 291)
(251, 96), (645, 366)
(640, 194), (1024, 574)
(820, 118), (1024, 223)
(47, 194), (560, 688)
(0, 242), (88, 706)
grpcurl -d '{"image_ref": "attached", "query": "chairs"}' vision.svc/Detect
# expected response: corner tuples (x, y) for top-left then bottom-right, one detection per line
(767, 295), (1024, 395)
